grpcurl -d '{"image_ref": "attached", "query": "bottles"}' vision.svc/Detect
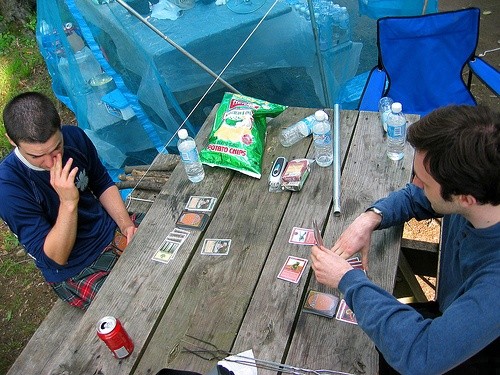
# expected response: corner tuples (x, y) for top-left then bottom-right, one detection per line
(177, 129), (205, 183)
(312, 110), (333, 167)
(387, 102), (406, 160)
(277, 112), (328, 147)
(379, 97), (395, 132)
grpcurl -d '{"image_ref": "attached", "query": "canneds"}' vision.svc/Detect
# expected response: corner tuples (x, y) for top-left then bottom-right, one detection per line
(96, 316), (134, 359)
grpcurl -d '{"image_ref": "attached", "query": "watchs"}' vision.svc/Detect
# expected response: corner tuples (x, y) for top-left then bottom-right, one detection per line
(367, 207), (385, 220)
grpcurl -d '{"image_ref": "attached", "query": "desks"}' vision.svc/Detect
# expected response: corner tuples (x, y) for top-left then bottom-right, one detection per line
(48, 103), (421, 375)
(75, 0), (292, 109)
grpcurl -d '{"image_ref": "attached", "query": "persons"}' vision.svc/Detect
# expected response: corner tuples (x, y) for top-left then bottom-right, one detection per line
(0, 91), (138, 311)
(310, 104), (500, 375)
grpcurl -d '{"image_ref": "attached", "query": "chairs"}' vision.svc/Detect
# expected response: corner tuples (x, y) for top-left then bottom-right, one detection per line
(358, 7), (500, 117)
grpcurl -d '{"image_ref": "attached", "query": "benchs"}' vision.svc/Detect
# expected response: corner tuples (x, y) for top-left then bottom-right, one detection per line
(53, 30), (136, 133)
(8, 155), (181, 375)
